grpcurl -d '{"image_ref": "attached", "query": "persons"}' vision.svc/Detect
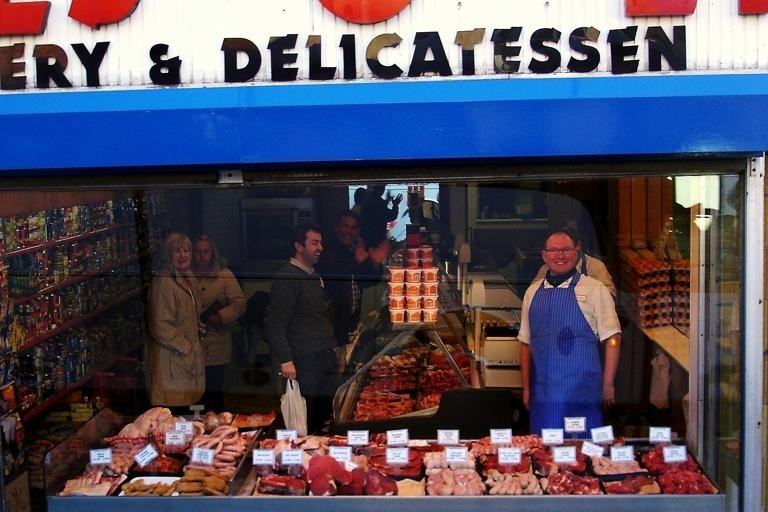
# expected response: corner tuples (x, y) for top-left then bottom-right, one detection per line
(185, 228), (248, 416)
(147, 232), (207, 415)
(268, 222), (338, 435)
(529, 227), (618, 303)
(516, 228), (624, 441)
(349, 184), (441, 247)
(315, 210), (392, 375)
(728, 293), (740, 373)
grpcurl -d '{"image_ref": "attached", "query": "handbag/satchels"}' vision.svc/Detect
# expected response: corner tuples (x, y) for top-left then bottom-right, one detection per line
(199, 321), (208, 341)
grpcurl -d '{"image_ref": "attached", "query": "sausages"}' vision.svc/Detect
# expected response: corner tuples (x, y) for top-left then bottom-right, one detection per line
(186, 425), (248, 479)
(83, 453), (135, 478)
(486, 469), (543, 496)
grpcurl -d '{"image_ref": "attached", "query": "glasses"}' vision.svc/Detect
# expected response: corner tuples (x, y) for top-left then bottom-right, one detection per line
(545, 246), (576, 253)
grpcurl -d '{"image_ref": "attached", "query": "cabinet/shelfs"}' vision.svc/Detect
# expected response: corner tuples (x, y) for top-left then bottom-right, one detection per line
(0, 190), (148, 421)
